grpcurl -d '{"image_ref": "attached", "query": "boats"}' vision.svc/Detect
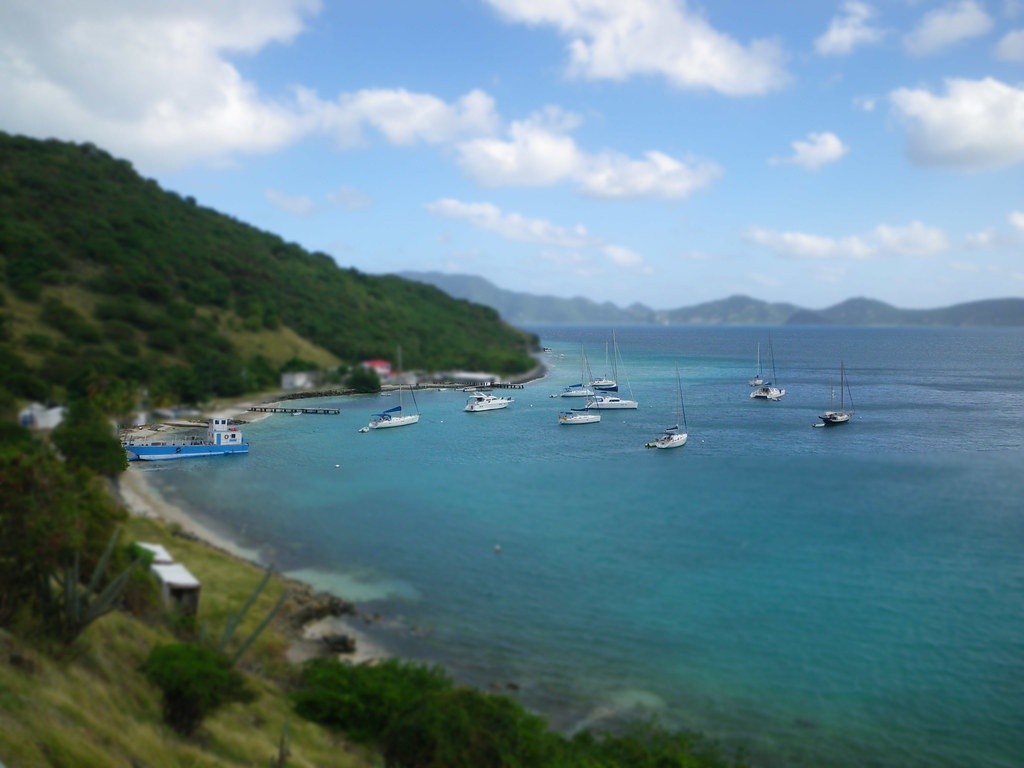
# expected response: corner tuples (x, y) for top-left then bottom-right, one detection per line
(748, 328), (785, 401)
(463, 390), (514, 411)
(645, 362), (687, 449)
(369, 343), (421, 429)
(557, 351), (601, 425)
(121, 417), (249, 462)
(560, 329), (638, 409)
(358, 427), (369, 432)
(818, 359), (855, 425)
